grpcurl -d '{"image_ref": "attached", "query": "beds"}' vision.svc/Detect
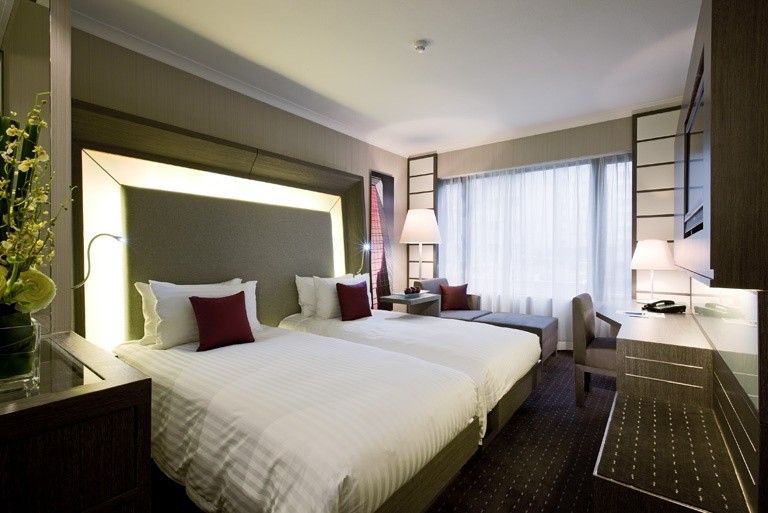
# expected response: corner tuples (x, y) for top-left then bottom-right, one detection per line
(115, 325), (485, 513)
(278, 309), (544, 449)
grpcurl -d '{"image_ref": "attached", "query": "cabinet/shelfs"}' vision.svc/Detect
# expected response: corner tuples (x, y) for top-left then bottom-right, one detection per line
(673, 1), (768, 292)
(1, 332), (152, 512)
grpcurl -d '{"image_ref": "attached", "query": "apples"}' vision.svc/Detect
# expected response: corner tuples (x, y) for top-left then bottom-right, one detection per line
(404, 286), (420, 294)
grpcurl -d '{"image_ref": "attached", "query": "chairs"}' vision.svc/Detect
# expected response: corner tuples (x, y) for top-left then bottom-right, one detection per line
(571, 293), (617, 407)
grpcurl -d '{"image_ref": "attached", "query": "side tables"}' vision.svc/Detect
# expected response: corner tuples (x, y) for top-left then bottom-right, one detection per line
(379, 294), (442, 317)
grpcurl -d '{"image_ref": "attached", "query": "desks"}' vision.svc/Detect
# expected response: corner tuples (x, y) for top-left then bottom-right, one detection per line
(594, 306), (717, 409)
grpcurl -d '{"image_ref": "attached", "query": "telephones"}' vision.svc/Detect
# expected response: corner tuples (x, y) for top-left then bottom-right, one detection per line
(641, 299), (687, 314)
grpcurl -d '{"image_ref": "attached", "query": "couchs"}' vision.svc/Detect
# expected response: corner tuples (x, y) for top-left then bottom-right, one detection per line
(336, 280), (373, 321)
(416, 276), (560, 366)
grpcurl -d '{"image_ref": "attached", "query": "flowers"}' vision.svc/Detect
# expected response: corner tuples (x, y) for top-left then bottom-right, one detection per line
(0, 86), (78, 380)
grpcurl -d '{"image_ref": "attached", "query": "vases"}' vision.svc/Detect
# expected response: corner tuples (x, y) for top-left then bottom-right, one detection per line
(0, 318), (42, 398)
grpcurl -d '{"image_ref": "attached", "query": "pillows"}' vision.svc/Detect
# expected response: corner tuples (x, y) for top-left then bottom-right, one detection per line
(439, 281), (471, 311)
(296, 274), (316, 318)
(313, 273), (371, 321)
(188, 291), (256, 352)
(135, 278), (244, 347)
(148, 279), (259, 349)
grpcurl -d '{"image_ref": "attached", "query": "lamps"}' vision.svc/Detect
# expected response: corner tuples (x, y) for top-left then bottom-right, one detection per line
(399, 209), (442, 279)
(71, 233), (124, 290)
(629, 239), (675, 302)
(355, 243), (370, 274)
